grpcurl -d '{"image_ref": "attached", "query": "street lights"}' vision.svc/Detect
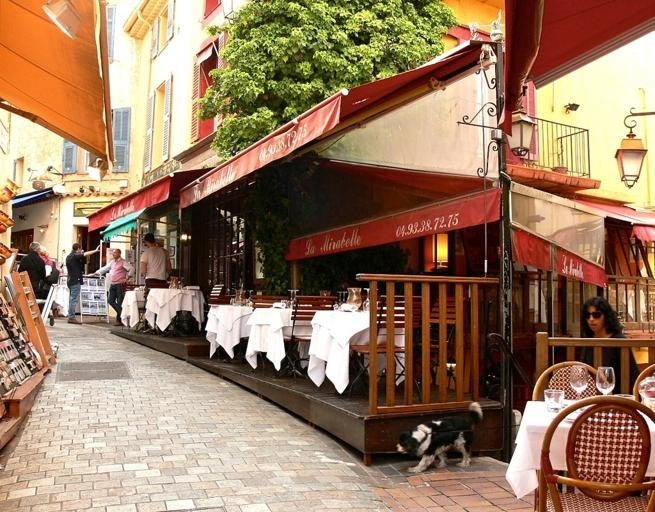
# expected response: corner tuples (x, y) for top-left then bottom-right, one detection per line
(471, 38), (528, 468)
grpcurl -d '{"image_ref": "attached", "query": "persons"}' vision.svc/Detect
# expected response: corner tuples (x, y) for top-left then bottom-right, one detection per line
(65, 242), (99, 323)
(580, 296), (640, 394)
(18, 242), (55, 327)
(88, 249), (135, 326)
(39, 246), (56, 290)
(140, 233), (172, 335)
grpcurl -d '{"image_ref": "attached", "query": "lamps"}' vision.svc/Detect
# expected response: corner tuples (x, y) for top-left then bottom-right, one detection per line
(433, 233), (448, 269)
(497, 85), (533, 156)
(614, 105), (655, 191)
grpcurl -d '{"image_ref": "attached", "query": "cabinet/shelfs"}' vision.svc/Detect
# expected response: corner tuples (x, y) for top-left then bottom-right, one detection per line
(10, 269), (59, 372)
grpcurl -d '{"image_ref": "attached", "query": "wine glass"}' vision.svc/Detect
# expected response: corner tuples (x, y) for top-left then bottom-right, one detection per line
(245, 290), (255, 307)
(363, 287), (379, 312)
(288, 289), (299, 308)
(569, 365), (588, 413)
(595, 366), (617, 396)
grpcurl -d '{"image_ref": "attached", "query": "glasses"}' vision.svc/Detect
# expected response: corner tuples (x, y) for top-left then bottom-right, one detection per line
(584, 312), (601, 318)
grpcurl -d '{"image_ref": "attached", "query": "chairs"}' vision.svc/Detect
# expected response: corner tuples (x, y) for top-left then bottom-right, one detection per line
(530, 359), (615, 403)
(632, 365), (654, 403)
(534, 393), (655, 512)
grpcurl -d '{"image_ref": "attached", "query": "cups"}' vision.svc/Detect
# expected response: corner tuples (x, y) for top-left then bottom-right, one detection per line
(544, 388), (564, 415)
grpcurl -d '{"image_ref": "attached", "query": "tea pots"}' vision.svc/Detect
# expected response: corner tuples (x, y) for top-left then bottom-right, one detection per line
(346, 287), (362, 308)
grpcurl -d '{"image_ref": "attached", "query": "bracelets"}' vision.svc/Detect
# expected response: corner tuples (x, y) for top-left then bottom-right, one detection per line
(126, 277), (129, 280)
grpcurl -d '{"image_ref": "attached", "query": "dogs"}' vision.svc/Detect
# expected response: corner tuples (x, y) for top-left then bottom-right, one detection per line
(396, 403), (484, 473)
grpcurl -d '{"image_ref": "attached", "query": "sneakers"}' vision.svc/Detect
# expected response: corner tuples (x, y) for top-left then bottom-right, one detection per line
(68, 319), (82, 324)
(142, 327), (151, 333)
(50, 315), (54, 326)
(112, 321), (124, 326)
